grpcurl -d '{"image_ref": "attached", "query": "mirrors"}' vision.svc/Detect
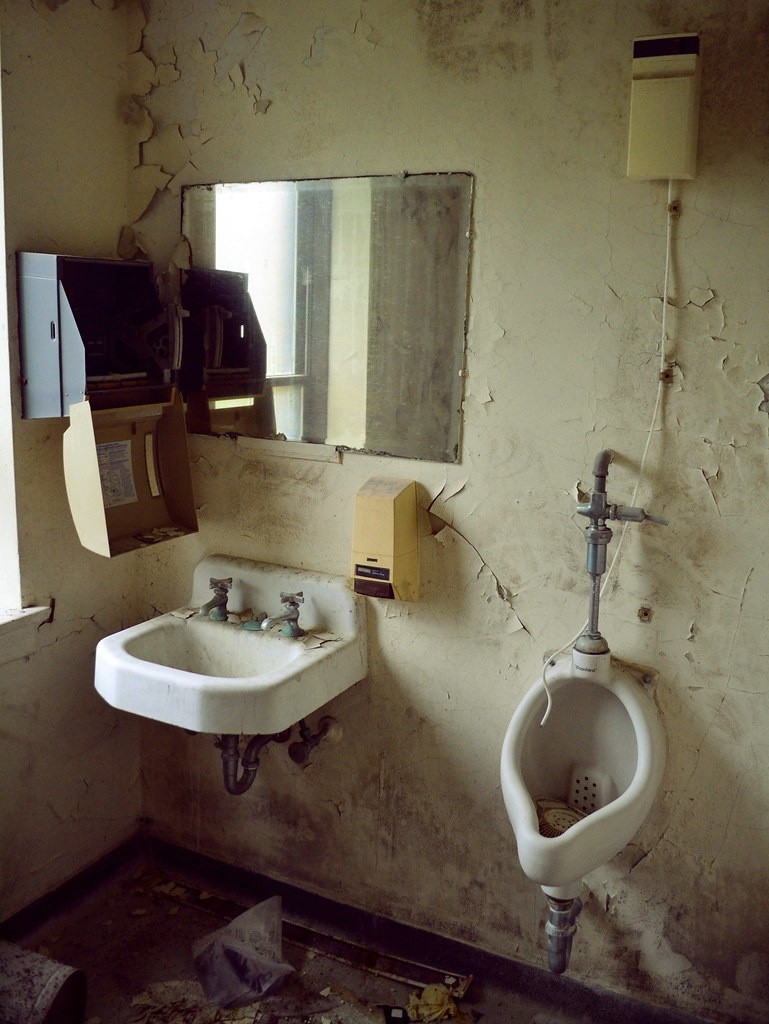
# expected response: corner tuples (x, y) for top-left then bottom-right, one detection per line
(173, 167), (476, 466)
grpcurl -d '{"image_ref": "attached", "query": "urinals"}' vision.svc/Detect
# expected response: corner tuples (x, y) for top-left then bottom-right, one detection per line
(498, 637), (668, 978)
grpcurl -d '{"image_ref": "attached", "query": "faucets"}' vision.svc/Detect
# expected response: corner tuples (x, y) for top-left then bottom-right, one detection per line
(197, 578), (306, 640)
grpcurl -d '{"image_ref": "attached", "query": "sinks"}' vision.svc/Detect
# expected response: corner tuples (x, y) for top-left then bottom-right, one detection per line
(94, 602), (350, 737)
